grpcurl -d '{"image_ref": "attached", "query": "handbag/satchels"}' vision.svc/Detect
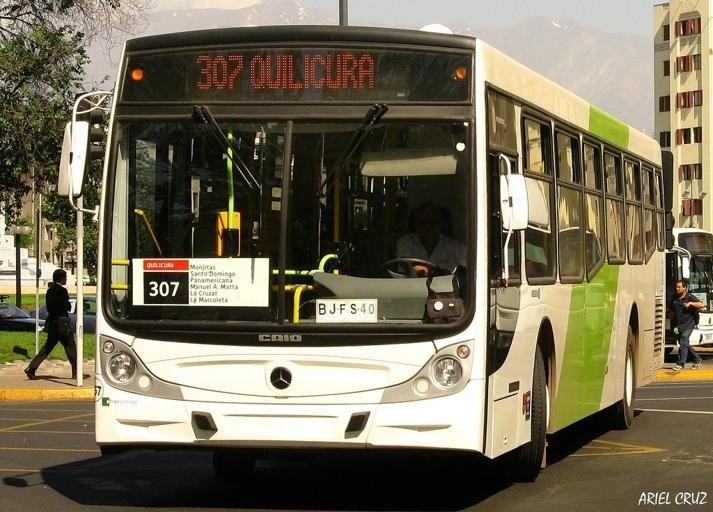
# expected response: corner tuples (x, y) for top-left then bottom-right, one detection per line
(43, 315), (71, 336)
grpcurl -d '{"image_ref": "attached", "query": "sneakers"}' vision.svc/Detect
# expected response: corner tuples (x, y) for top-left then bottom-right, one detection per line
(688, 359), (702, 369)
(673, 364), (683, 371)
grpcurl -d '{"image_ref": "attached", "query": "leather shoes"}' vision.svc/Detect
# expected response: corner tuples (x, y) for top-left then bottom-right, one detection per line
(72, 374), (90, 379)
(24, 366), (37, 380)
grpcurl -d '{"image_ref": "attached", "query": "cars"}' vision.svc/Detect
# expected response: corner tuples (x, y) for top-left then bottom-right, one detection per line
(0, 303), (46, 333)
(28, 295), (96, 335)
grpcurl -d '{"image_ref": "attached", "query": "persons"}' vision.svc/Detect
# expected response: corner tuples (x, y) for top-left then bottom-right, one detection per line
(23, 268), (91, 381)
(395, 201), (467, 275)
(667, 279), (706, 372)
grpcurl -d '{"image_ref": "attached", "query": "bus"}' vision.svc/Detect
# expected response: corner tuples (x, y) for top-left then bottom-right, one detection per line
(49, 24), (665, 483)
(664, 225), (713, 352)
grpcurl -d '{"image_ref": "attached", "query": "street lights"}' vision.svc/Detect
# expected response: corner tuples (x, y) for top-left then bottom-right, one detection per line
(6, 218), (31, 309)
(681, 190), (706, 228)
